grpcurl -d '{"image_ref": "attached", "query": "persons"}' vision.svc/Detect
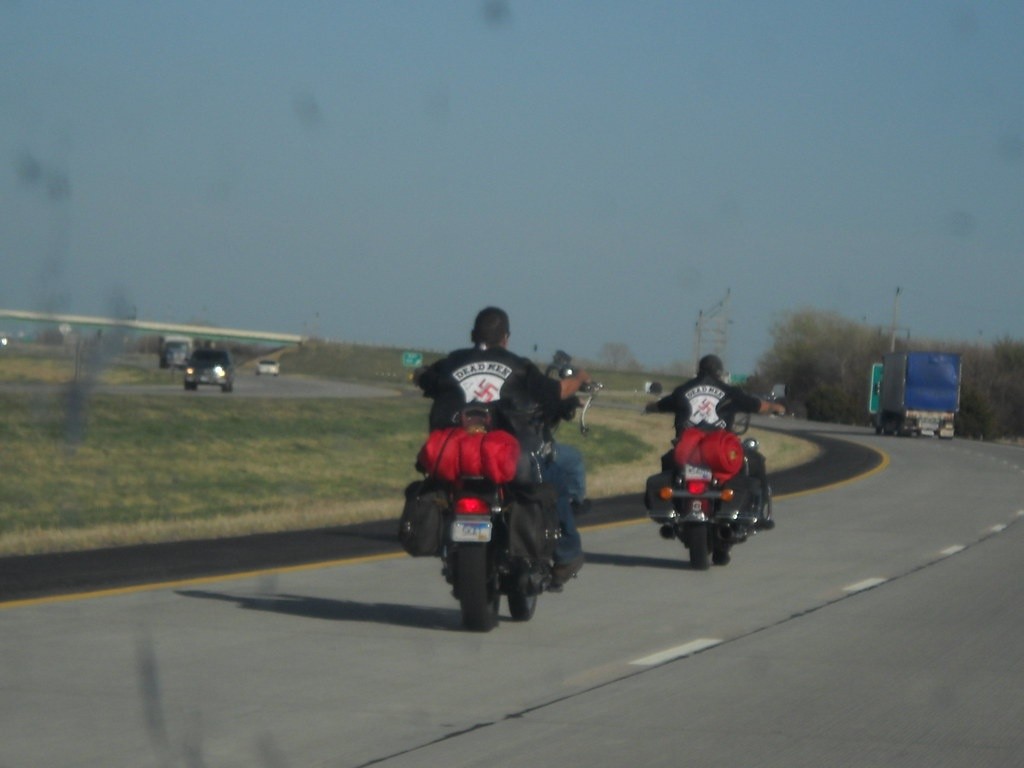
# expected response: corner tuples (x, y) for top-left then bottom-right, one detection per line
(644, 354), (785, 531)
(414, 306), (590, 586)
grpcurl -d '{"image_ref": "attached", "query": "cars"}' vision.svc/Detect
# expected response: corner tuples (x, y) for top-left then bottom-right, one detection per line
(257, 360), (280, 376)
(185, 348), (234, 393)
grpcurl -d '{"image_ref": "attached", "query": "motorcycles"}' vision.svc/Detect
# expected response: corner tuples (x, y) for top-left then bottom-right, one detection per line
(642, 380), (796, 571)
(401, 350), (604, 633)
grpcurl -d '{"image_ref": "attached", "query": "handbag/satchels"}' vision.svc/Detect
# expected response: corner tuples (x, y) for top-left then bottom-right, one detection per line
(398, 477), (452, 556)
(508, 499), (566, 556)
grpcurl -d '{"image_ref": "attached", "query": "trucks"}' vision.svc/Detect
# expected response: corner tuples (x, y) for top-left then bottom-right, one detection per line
(874, 350), (963, 440)
(159, 336), (193, 370)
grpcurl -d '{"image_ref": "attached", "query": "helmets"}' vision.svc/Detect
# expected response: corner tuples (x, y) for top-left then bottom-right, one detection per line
(472, 306), (510, 343)
(699, 354), (723, 378)
